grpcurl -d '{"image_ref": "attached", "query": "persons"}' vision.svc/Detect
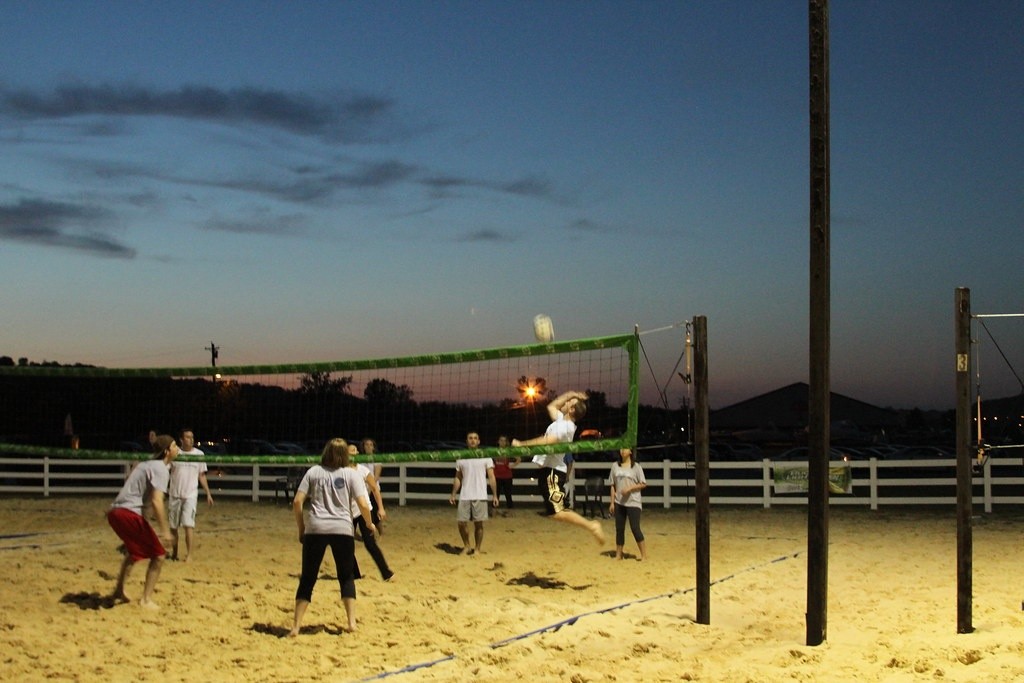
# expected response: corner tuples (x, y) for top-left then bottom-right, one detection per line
(107, 435), (179, 609)
(450, 431), (499, 556)
(512, 390), (606, 546)
(286, 438), (377, 637)
(491, 435), (521, 510)
(150, 429), (214, 562)
(345, 438), (395, 582)
(609, 449), (649, 562)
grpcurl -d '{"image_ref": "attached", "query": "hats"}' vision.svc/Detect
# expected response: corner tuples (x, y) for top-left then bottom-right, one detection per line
(152, 434), (174, 459)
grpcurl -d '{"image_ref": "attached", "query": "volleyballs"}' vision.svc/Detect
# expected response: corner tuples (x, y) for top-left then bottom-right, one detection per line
(532, 313), (554, 343)
